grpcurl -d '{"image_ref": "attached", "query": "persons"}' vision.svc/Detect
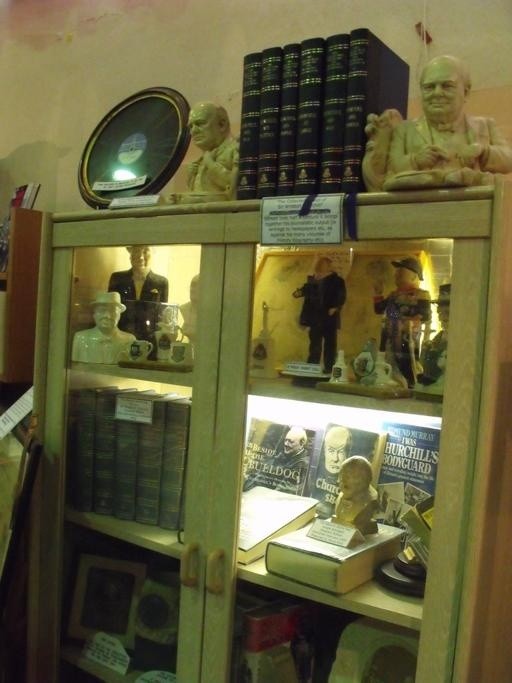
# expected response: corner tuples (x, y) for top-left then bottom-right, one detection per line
(383, 54), (511, 190)
(371, 257), (429, 382)
(324, 427), (351, 474)
(105, 245), (168, 360)
(182, 101), (240, 197)
(382, 490), (388, 513)
(408, 493), (417, 505)
(263, 428), (310, 484)
(290, 618), (315, 683)
(371, 257), (429, 389)
(70, 290), (136, 364)
(334, 455), (380, 521)
(291, 257), (348, 373)
(237, 657), (254, 683)
(180, 272), (200, 342)
(392, 507), (402, 526)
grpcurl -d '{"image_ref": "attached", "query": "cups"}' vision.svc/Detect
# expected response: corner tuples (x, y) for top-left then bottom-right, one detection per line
(127, 338), (155, 361)
(169, 341), (193, 364)
(155, 330), (177, 361)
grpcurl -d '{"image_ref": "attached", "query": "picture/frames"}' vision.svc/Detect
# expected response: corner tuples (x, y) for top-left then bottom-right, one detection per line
(60, 541), (154, 659)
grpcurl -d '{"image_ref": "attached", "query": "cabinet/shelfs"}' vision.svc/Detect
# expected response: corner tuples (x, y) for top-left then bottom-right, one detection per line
(25, 173), (512, 683)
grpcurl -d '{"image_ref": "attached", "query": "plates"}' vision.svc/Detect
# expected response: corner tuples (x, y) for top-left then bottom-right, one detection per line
(118, 359), (194, 372)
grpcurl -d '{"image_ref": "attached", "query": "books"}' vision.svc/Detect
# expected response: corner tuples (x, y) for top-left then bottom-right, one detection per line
(8, 181), (42, 220)
(235, 599), (319, 683)
(306, 422), (389, 518)
(244, 418), (323, 495)
(233, 27), (411, 201)
(66, 387), (191, 531)
(377, 422), (439, 529)
(235, 486), (320, 567)
(265, 516), (407, 595)
